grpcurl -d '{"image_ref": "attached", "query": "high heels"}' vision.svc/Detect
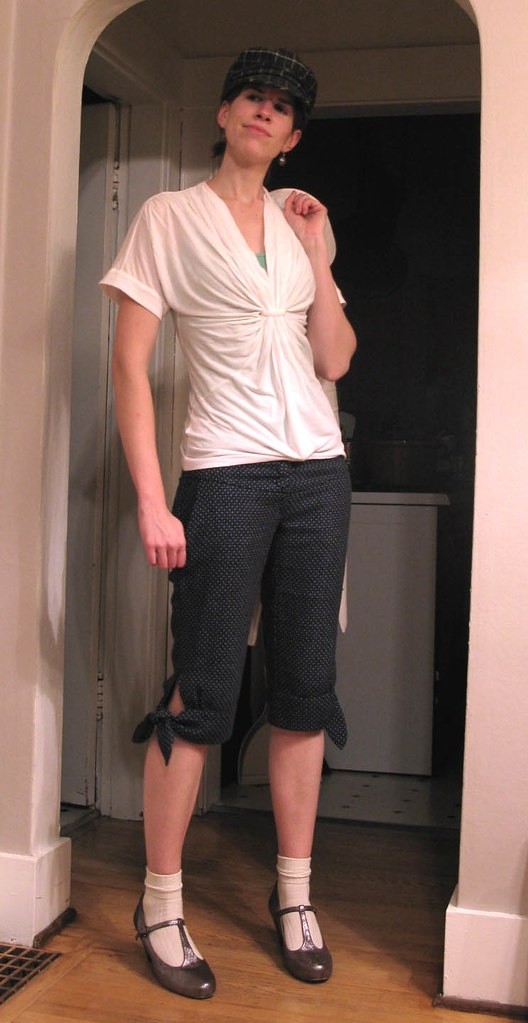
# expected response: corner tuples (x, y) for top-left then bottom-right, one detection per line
(268, 880), (333, 981)
(133, 892), (216, 998)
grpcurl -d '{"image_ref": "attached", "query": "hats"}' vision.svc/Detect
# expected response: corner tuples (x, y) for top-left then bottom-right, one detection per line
(222, 46), (318, 127)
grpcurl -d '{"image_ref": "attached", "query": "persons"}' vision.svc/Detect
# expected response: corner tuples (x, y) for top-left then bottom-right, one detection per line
(99, 47), (356, 1001)
